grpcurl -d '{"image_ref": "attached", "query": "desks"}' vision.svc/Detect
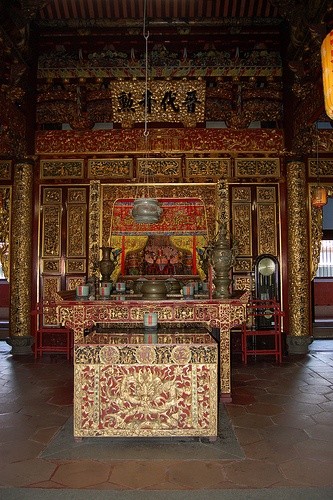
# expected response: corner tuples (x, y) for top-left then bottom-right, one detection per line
(74, 335), (218, 443)
(29, 300), (246, 404)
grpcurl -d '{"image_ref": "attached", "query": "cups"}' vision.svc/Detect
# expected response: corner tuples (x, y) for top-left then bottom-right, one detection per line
(76, 285), (89, 297)
(115, 283), (125, 293)
(99, 287), (110, 298)
(183, 286), (194, 296)
(144, 314), (157, 330)
(102, 283), (112, 291)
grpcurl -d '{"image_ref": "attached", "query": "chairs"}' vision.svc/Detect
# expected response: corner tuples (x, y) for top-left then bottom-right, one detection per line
(242, 299), (285, 363)
(31, 303), (73, 360)
